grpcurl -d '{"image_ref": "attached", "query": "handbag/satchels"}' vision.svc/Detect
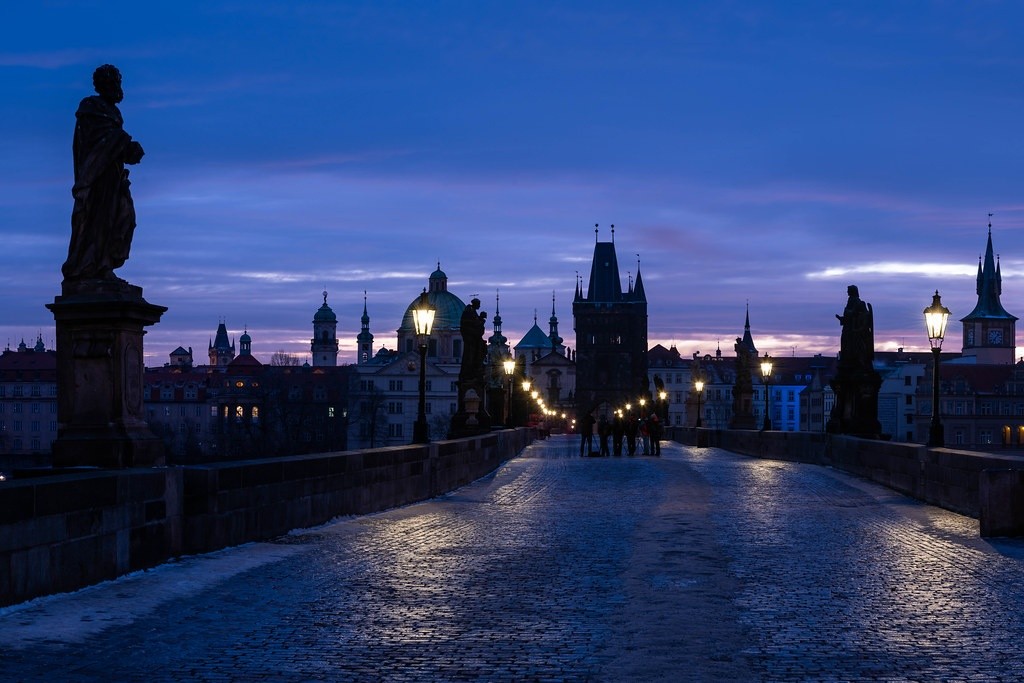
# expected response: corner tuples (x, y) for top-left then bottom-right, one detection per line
(588, 452), (599, 457)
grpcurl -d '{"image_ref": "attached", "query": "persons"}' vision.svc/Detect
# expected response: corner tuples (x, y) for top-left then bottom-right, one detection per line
(457, 298), (488, 409)
(835, 285), (875, 373)
(734, 338), (752, 363)
(61, 64), (143, 298)
(689, 354), (701, 372)
(535, 418), (551, 440)
(578, 408), (663, 457)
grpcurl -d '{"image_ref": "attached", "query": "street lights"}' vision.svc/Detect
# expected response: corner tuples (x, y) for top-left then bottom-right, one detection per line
(923, 290), (952, 448)
(522, 378), (531, 427)
(694, 378), (704, 428)
(613, 396), (649, 425)
(502, 353), (516, 429)
(409, 286), (437, 444)
(659, 389), (668, 426)
(531, 390), (577, 429)
(759, 358), (774, 430)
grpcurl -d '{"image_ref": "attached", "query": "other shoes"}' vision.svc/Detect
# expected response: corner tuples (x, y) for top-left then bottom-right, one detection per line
(650, 451), (654, 456)
(642, 453), (649, 456)
(606, 454), (610, 456)
(656, 450), (660, 456)
(613, 454), (621, 456)
(602, 454), (605, 456)
(579, 454), (583, 457)
